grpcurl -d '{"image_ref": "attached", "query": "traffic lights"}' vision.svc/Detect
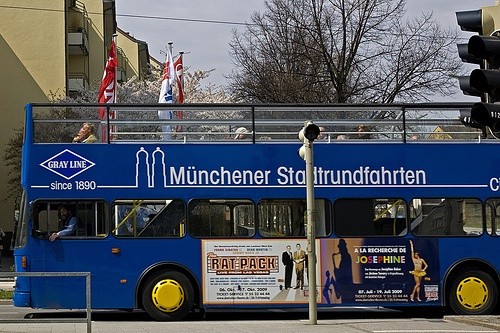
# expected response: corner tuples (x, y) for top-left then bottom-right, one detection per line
(298, 119), (321, 160)
(454, 5), (499, 138)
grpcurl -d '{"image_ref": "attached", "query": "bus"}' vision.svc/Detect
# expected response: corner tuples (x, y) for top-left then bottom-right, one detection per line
(12, 103), (498, 321)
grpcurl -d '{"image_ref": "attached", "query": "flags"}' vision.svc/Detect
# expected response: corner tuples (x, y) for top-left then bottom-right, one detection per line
(97, 41), (118, 143)
(158, 43), (176, 139)
(173, 55), (184, 121)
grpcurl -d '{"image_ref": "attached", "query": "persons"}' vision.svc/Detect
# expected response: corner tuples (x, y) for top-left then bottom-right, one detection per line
(282, 245), (294, 291)
(292, 244), (306, 291)
(408, 239), (428, 302)
(303, 246), (317, 285)
(49, 206), (78, 242)
(233, 122), (423, 139)
(72, 122), (99, 143)
